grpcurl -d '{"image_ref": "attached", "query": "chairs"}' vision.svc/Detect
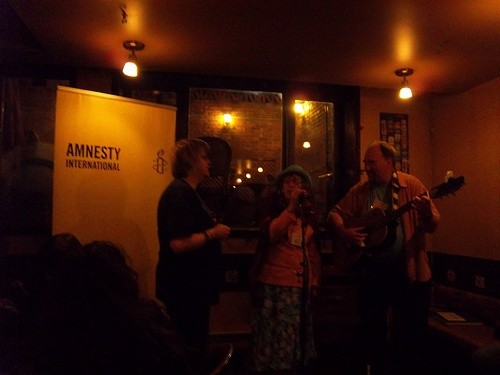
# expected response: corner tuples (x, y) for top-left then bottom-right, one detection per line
(209, 341), (234, 375)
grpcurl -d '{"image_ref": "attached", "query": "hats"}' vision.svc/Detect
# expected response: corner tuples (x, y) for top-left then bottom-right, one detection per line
(276, 164), (312, 187)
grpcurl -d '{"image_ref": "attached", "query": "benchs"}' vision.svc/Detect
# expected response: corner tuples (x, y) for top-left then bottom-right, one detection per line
(427, 282), (500, 375)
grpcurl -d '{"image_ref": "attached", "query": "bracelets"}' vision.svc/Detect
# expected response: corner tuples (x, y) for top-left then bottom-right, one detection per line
(201, 231), (210, 244)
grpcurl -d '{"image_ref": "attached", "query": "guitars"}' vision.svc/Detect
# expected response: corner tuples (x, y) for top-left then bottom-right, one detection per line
(348, 174), (466, 252)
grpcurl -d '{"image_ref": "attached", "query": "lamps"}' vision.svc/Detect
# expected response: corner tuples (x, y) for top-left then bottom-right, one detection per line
(122, 40), (145, 77)
(394, 69), (414, 100)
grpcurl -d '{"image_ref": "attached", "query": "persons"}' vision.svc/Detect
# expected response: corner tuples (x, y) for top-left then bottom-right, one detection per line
(248, 165), (353, 375)
(327, 141), (440, 375)
(154, 138), (231, 375)
(0, 234), (195, 375)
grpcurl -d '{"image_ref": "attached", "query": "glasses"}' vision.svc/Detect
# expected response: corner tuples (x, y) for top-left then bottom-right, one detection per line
(283, 179), (304, 185)
